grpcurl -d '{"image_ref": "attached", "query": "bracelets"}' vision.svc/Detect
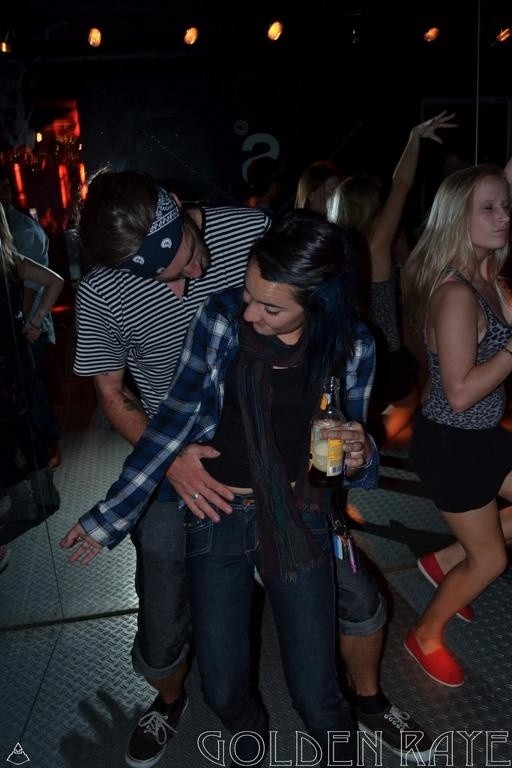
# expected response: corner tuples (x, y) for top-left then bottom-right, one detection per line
(502, 345), (512, 356)
(29, 321), (42, 330)
(358, 459), (371, 469)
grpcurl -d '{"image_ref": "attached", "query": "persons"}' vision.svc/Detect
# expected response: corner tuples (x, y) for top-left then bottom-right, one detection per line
(1, 161), (60, 471)
(237, 152), (471, 266)
(323, 108), (461, 531)
(1, 199), (68, 575)
(57, 208), (383, 768)
(72, 169), (436, 768)
(392, 154), (511, 690)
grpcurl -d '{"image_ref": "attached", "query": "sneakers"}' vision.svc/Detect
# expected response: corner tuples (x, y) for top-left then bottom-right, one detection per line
(418, 552), (473, 623)
(356, 702), (435, 763)
(125, 689), (189, 767)
(404, 627), (465, 687)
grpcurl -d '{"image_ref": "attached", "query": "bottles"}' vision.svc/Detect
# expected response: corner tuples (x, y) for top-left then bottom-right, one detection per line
(308, 376), (347, 489)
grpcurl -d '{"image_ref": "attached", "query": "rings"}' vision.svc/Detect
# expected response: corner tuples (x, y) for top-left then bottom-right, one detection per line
(191, 492), (201, 500)
(79, 536), (84, 541)
